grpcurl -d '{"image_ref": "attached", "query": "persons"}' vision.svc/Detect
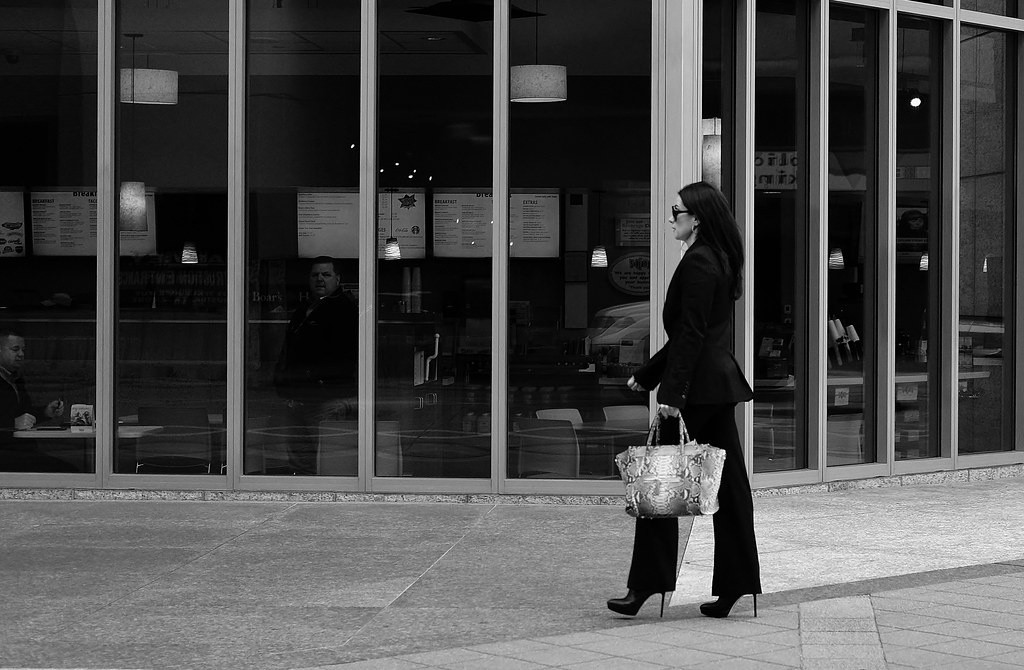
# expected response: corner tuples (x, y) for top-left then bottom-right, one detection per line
(0, 330), (79, 472)
(607, 181), (764, 618)
(279, 257), (359, 476)
(897, 210), (928, 239)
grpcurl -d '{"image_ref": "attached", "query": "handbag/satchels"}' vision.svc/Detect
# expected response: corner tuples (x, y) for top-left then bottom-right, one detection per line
(615, 409), (727, 518)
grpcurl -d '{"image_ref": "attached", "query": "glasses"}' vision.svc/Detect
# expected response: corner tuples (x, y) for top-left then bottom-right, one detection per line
(672, 205), (689, 222)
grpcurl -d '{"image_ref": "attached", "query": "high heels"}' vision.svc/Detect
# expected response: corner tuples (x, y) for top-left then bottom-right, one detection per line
(607, 588), (665, 617)
(700, 584), (757, 618)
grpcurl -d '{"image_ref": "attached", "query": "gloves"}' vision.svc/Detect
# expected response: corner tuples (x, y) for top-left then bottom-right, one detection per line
(660, 405), (679, 420)
(627, 375), (645, 391)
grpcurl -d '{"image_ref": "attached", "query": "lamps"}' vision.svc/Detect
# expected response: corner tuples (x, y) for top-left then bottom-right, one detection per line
(180, 192), (200, 263)
(119, 55), (179, 105)
(591, 190), (608, 267)
(118, 34), (148, 231)
(918, 200), (929, 271)
(829, 195), (844, 270)
(905, 87), (923, 109)
(384, 188), (401, 261)
(510, 0), (568, 103)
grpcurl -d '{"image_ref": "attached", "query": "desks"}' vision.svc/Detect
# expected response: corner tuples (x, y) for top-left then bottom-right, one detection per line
(12, 423), (163, 475)
(753, 369), (992, 460)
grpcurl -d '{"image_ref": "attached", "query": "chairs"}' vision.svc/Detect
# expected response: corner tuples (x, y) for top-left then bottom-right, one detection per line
(315, 419), (404, 478)
(513, 405), (653, 480)
(135, 404), (212, 476)
